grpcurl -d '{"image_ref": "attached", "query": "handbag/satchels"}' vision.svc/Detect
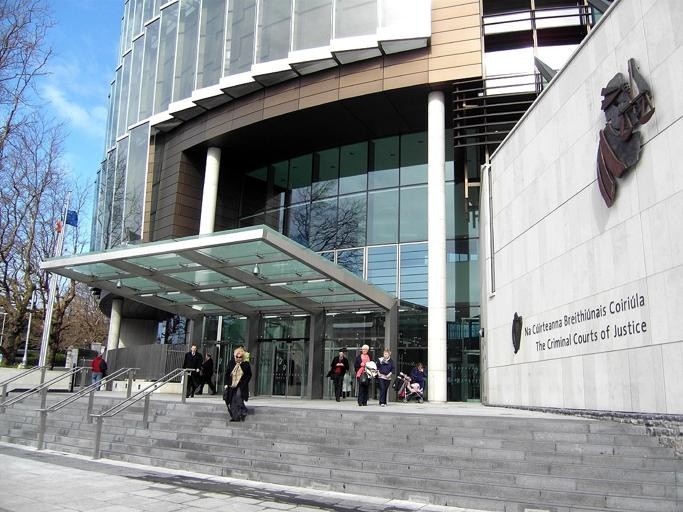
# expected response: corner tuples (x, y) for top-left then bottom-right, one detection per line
(223, 390), (228, 400)
(101, 360), (107, 370)
(360, 372), (369, 386)
(373, 376), (380, 384)
(327, 368), (335, 380)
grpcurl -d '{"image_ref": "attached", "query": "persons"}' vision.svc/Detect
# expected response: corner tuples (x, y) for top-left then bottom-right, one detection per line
(182, 343), (204, 399)
(194, 352), (218, 396)
(222, 346), (252, 422)
(326, 343), (427, 408)
(91, 354), (107, 391)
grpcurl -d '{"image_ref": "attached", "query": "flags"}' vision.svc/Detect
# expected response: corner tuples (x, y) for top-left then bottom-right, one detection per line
(60, 206), (79, 227)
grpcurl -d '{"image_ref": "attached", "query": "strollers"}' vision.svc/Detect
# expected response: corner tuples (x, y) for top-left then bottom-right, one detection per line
(392, 371), (424, 404)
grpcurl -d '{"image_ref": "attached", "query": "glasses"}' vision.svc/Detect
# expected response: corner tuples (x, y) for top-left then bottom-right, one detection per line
(236, 356), (242, 358)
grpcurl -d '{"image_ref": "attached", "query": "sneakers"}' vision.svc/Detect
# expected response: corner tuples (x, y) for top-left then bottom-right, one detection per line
(359, 399), (367, 405)
(211, 390), (218, 395)
(336, 395), (340, 402)
(186, 392), (194, 397)
(195, 392), (202, 394)
(381, 404), (387, 407)
